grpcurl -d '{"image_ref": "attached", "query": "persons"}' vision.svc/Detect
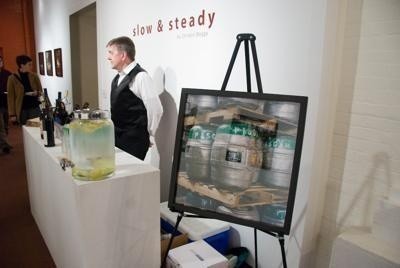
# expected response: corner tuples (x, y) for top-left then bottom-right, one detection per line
(0, 56), (14, 135)
(6, 54), (45, 127)
(104, 35), (164, 162)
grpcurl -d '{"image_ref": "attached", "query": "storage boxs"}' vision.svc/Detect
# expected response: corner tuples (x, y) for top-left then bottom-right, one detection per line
(160, 202), (237, 268)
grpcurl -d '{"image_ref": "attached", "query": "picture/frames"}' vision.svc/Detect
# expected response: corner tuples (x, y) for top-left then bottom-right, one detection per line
(38, 48), (63, 77)
(169, 87), (308, 236)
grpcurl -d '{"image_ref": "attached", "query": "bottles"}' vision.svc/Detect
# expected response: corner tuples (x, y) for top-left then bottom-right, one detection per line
(68, 109), (115, 180)
(40, 88), (65, 147)
